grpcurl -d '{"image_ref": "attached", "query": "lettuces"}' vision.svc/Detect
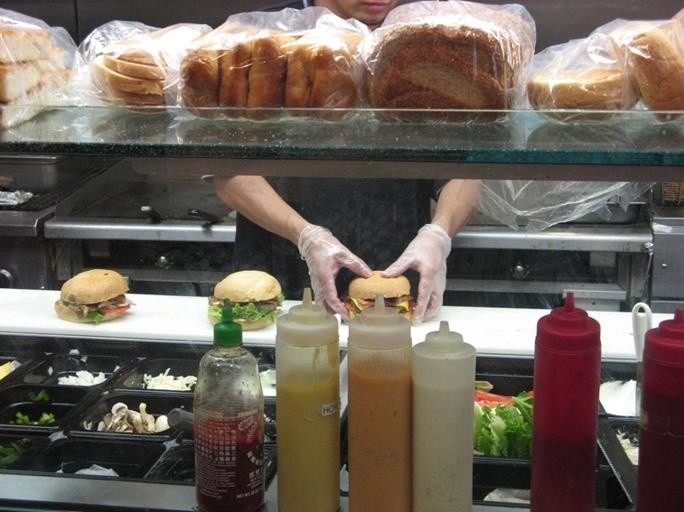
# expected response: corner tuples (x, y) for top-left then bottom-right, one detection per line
(473, 391), (533, 457)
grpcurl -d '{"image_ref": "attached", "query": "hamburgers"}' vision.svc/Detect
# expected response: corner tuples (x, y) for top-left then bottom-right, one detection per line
(344, 270), (416, 327)
(54, 268), (137, 324)
(207, 270), (285, 331)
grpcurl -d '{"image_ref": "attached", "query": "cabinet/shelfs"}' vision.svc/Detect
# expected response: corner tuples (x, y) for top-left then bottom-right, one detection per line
(0, 109), (682, 511)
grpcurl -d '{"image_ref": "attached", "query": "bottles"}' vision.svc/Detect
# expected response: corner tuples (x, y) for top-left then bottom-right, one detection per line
(272, 286), (341, 509)
(348, 294), (413, 510)
(637, 310), (684, 510)
(531, 289), (603, 512)
(190, 295), (266, 511)
(413, 317), (474, 512)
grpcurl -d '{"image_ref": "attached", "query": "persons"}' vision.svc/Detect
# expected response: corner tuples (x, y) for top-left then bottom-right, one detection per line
(210, 0), (487, 331)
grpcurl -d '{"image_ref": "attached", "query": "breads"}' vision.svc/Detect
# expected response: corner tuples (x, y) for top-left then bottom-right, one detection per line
(0, 0), (683, 135)
